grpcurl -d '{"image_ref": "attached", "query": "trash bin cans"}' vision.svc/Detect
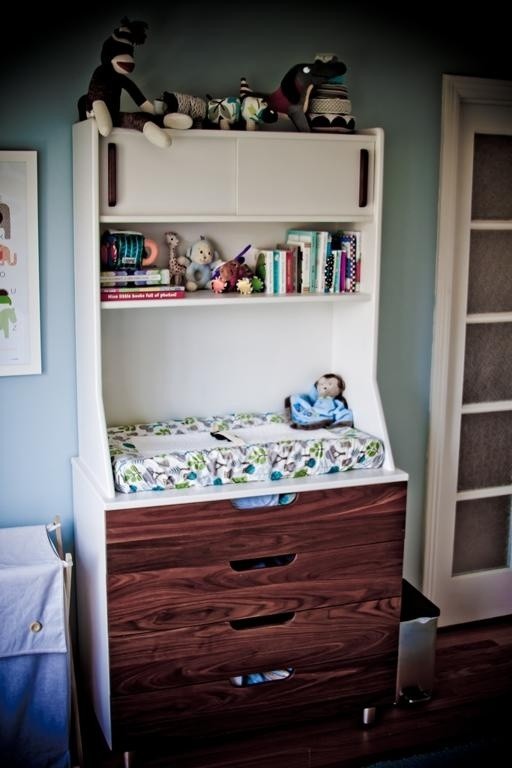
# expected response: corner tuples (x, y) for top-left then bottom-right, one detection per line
(396, 577), (440, 702)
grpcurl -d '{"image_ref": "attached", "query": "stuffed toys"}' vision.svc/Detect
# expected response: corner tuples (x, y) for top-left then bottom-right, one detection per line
(77, 16), (358, 148)
(165, 232), (265, 295)
(284, 374), (353, 430)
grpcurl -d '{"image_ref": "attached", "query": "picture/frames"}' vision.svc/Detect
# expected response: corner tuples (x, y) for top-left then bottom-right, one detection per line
(0, 150), (44, 377)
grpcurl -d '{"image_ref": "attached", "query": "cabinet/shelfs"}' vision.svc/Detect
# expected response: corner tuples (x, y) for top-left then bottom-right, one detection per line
(0, 514), (73, 768)
(70, 117), (410, 768)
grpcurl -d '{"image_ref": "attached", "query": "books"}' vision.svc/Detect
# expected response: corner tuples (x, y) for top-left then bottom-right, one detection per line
(252, 229), (361, 294)
(100, 267), (185, 301)
